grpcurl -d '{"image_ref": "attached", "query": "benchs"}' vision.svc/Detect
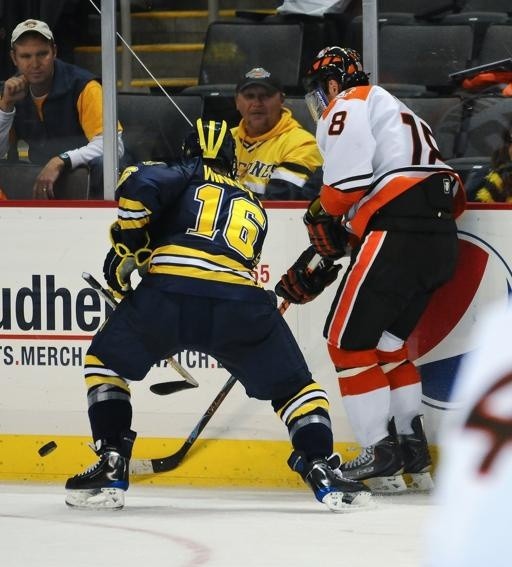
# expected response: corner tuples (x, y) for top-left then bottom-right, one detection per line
(72, 1), (277, 96)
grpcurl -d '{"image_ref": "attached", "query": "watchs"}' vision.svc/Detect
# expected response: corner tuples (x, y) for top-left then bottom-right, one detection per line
(57, 153), (72, 170)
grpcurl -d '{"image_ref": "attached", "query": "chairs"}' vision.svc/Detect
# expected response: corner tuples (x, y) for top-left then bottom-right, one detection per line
(445, 156), (492, 202)
(118, 93), (204, 171)
(282, 96), (317, 133)
(470, 22), (512, 97)
(180, 19), (304, 106)
(458, 93), (512, 160)
(399, 94), (469, 167)
(347, 0), (512, 65)
(382, 22), (476, 98)
(0, 162), (89, 200)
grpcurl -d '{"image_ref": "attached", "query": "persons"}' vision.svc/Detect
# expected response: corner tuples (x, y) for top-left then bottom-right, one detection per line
(0, 18), (124, 200)
(275, 44), (467, 498)
(63, 116), (374, 512)
(228, 66), (324, 200)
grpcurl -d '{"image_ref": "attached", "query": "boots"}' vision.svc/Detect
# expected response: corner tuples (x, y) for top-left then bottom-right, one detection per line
(340, 415), (431, 481)
(287, 450), (370, 503)
(65, 430), (137, 491)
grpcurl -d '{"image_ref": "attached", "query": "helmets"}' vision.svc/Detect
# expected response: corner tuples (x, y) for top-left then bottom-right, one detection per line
(179, 118), (237, 180)
(300, 45), (369, 125)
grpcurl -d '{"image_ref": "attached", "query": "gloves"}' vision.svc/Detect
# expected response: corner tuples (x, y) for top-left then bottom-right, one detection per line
(275, 245), (342, 304)
(303, 196), (349, 261)
(103, 221), (153, 300)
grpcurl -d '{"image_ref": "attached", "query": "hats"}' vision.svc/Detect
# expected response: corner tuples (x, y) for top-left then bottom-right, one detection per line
(236, 67), (284, 94)
(10, 18), (55, 49)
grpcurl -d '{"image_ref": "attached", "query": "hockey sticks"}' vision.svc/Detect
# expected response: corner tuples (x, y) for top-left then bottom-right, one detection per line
(83, 274), (198, 395)
(127, 245), (319, 474)
